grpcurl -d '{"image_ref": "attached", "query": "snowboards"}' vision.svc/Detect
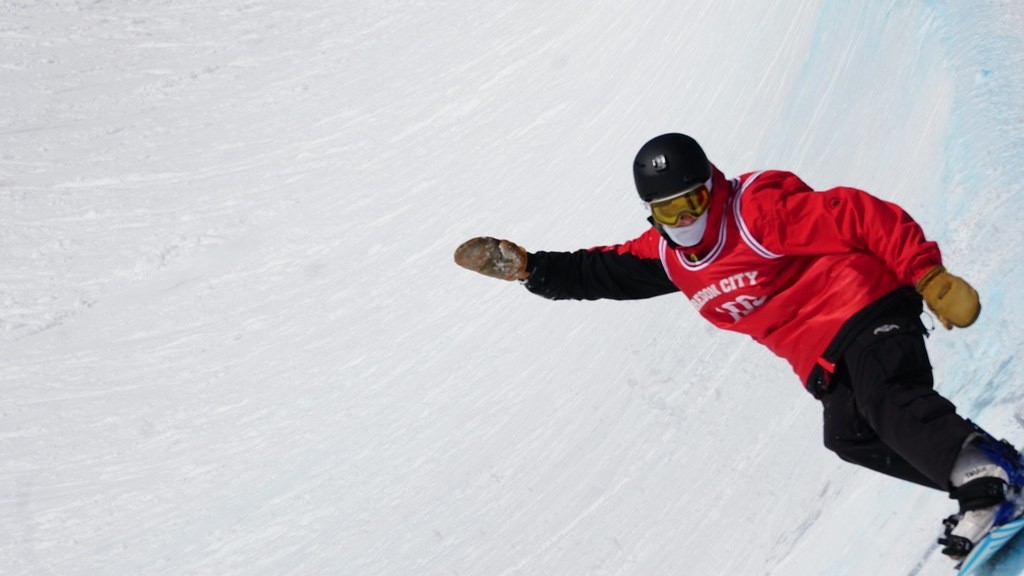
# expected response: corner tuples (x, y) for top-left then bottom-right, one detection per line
(958, 513), (1024, 576)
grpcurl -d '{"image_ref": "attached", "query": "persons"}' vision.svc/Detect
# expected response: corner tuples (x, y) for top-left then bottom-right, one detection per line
(454, 133), (1024, 557)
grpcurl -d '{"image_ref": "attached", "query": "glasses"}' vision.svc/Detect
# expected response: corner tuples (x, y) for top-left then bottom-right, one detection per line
(650, 185), (711, 226)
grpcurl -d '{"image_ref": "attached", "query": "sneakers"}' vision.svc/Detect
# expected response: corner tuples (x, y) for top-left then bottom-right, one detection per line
(941, 429), (1023, 558)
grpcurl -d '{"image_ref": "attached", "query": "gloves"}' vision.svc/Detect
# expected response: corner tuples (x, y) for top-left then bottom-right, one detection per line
(918, 265), (982, 331)
(454, 237), (533, 282)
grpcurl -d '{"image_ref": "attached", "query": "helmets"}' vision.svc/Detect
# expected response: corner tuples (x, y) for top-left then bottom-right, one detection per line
(634, 133), (712, 202)
